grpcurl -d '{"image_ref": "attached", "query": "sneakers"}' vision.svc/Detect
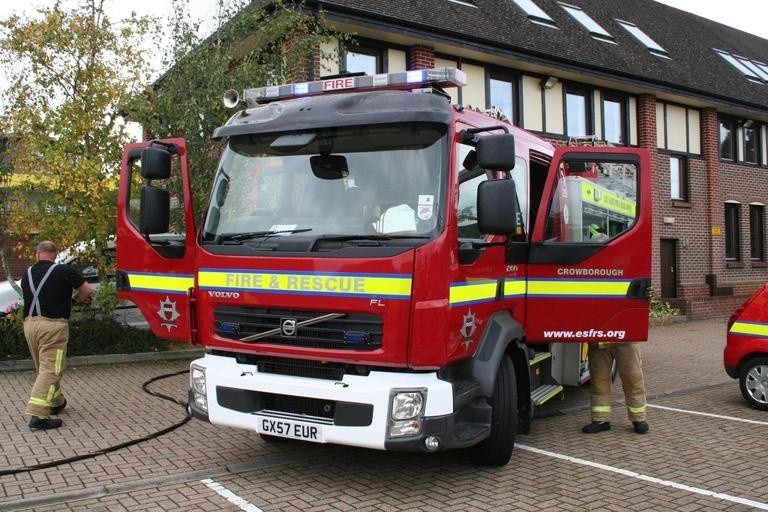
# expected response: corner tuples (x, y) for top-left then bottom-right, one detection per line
(49, 398), (67, 415)
(634, 419), (650, 433)
(583, 420), (612, 435)
(28, 414), (62, 430)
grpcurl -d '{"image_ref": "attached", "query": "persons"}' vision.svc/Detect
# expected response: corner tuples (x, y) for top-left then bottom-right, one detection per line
(20, 240), (93, 429)
(563, 232), (656, 435)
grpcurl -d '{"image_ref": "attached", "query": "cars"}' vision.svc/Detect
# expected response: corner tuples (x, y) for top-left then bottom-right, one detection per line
(0, 230), (195, 332)
(723, 279), (768, 412)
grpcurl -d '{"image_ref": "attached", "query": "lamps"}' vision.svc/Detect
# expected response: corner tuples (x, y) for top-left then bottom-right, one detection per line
(539, 75), (558, 89)
(739, 118), (754, 129)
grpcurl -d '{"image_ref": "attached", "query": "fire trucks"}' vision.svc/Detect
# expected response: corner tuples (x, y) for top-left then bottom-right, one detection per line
(114, 67), (653, 467)
